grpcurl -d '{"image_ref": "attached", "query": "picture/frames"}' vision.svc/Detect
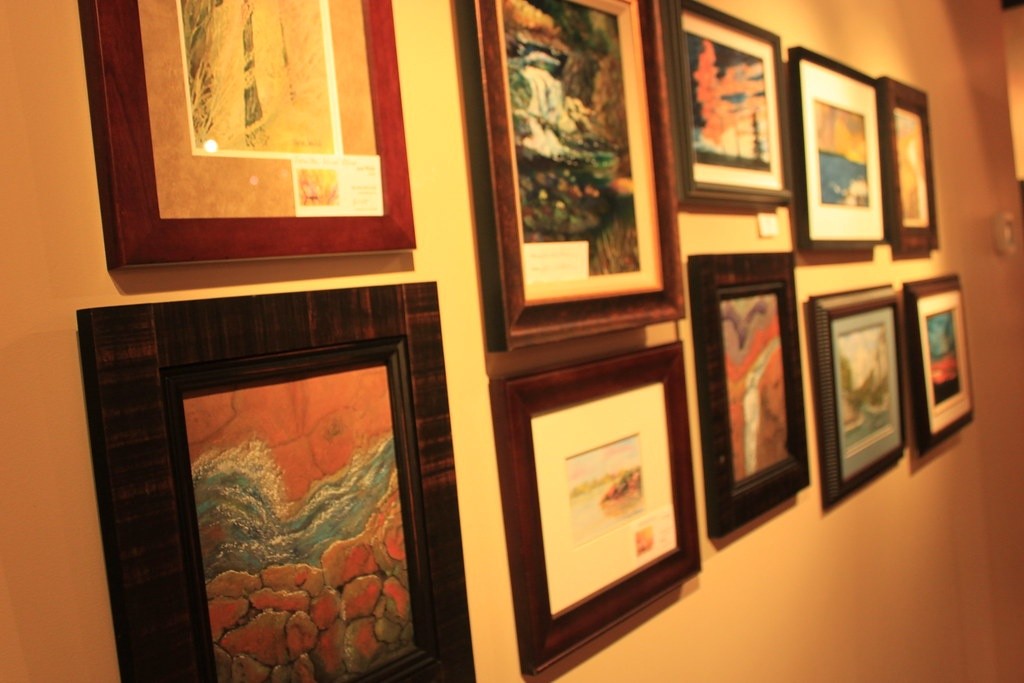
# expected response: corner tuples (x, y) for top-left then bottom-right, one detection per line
(806, 282), (908, 513)
(685, 251), (812, 538)
(454, 0), (687, 353)
(788, 45), (894, 253)
(902, 273), (974, 459)
(77, 280), (478, 683)
(76, 0), (416, 272)
(875, 75), (940, 257)
(659, 0), (792, 208)
(487, 340), (703, 676)
